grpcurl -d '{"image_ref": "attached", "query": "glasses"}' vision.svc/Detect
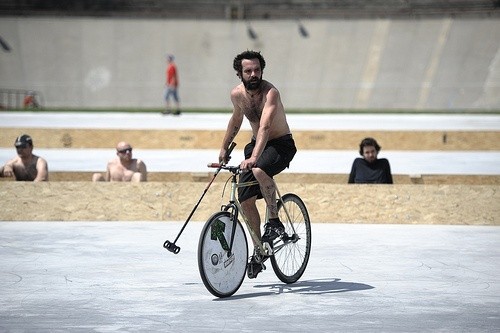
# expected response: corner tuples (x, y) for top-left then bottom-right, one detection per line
(15, 143), (27, 148)
(118, 147), (132, 153)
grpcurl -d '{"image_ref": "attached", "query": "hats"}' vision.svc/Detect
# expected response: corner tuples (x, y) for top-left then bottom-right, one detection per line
(15, 134), (32, 146)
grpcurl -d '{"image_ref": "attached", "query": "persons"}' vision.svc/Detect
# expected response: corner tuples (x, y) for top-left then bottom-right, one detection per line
(0, 134), (49, 182)
(162, 53), (181, 115)
(217, 50), (297, 279)
(92, 140), (147, 182)
(348, 137), (393, 184)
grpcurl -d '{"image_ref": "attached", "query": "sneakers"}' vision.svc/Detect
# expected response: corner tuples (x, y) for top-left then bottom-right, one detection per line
(261, 221), (285, 242)
(247, 255), (264, 279)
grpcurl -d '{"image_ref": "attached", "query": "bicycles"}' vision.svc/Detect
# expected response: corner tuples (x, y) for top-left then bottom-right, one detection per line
(197, 163), (312, 296)
